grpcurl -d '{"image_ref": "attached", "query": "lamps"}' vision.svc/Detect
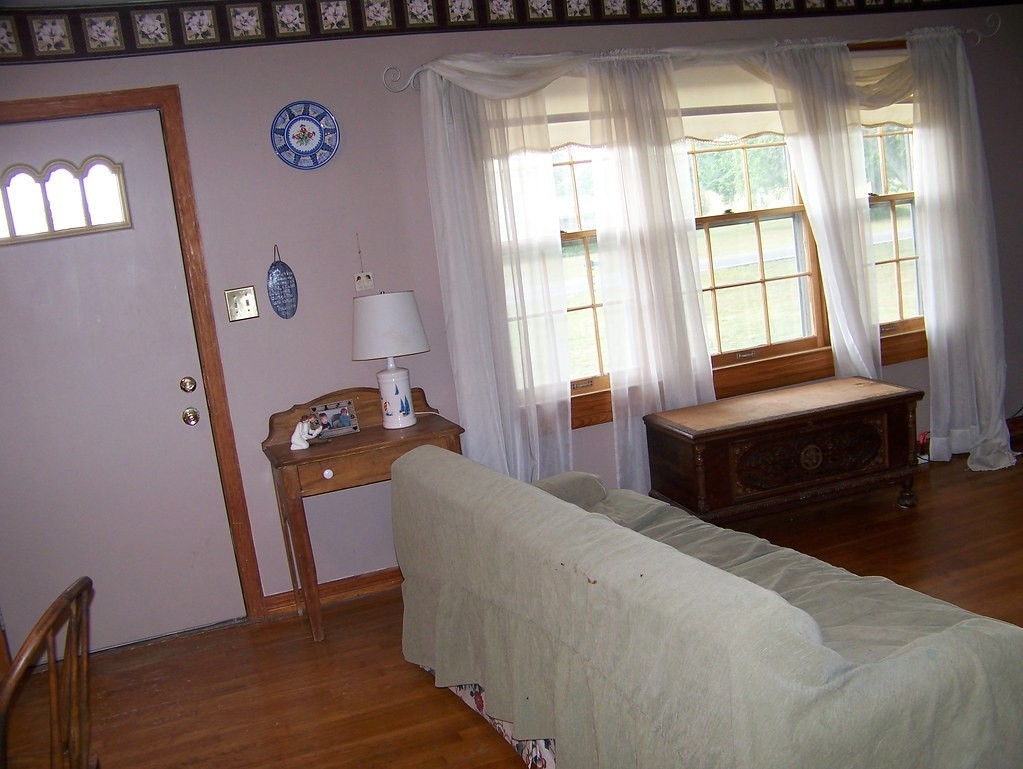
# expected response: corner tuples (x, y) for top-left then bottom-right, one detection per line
(351, 290), (430, 429)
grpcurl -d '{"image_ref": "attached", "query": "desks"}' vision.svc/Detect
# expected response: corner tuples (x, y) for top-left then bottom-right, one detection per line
(262, 386), (466, 643)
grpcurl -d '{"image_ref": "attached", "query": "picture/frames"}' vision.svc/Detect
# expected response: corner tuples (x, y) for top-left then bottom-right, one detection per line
(309, 398), (360, 438)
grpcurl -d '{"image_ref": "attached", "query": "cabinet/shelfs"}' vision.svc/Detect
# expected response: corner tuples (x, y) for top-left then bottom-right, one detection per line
(643, 377), (925, 525)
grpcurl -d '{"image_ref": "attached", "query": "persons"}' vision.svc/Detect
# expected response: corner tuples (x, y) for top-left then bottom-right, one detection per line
(333, 408), (350, 429)
(290, 414), (323, 450)
(319, 412), (336, 431)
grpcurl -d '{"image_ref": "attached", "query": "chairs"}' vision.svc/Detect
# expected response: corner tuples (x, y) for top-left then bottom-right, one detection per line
(0, 577), (96, 769)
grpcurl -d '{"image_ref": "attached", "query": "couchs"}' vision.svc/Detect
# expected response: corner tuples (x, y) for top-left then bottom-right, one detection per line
(392, 446), (1023, 769)
